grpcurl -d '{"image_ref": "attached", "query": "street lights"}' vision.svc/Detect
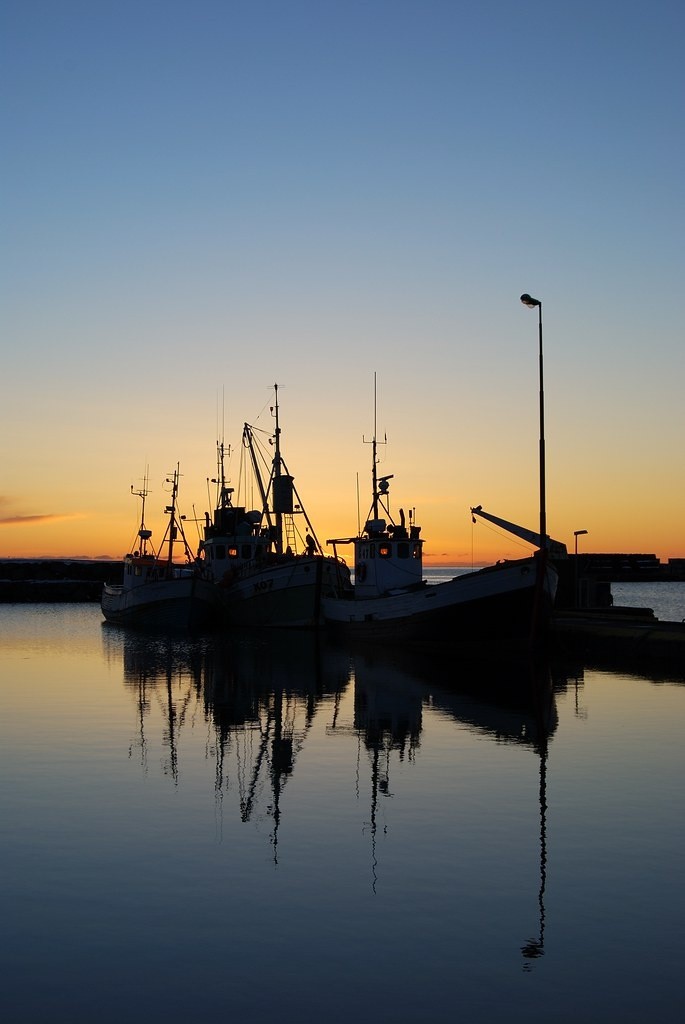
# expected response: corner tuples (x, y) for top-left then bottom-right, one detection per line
(519, 291), (546, 558)
(574, 531), (588, 609)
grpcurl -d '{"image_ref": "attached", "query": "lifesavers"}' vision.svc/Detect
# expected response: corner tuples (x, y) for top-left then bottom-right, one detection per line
(355, 560), (367, 582)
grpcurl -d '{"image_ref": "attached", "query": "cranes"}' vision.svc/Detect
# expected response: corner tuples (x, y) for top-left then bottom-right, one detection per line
(472, 505), (571, 573)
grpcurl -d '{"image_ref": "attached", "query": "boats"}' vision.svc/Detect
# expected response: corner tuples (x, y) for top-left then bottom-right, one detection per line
(316, 370), (563, 649)
(191, 384), (352, 628)
(100, 465), (194, 632)
